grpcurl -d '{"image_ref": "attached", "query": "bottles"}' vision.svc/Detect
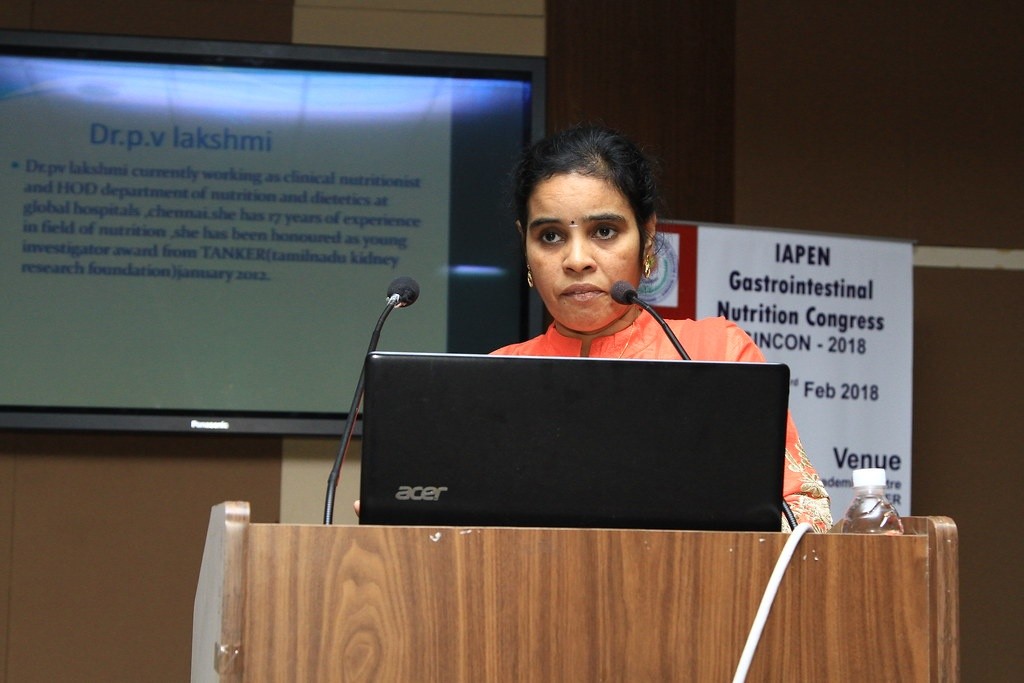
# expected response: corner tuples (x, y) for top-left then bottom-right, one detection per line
(842, 468), (904, 537)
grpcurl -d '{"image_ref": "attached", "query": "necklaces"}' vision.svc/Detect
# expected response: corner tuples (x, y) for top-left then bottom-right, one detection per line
(618, 322), (634, 358)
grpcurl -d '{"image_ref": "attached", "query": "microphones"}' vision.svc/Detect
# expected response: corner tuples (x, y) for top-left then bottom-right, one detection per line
(609, 280), (798, 531)
(323, 277), (421, 527)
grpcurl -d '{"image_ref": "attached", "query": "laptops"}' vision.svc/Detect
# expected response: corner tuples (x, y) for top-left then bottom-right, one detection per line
(357, 350), (791, 533)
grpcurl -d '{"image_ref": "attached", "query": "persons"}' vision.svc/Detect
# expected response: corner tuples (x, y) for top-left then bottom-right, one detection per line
(352, 126), (833, 534)
